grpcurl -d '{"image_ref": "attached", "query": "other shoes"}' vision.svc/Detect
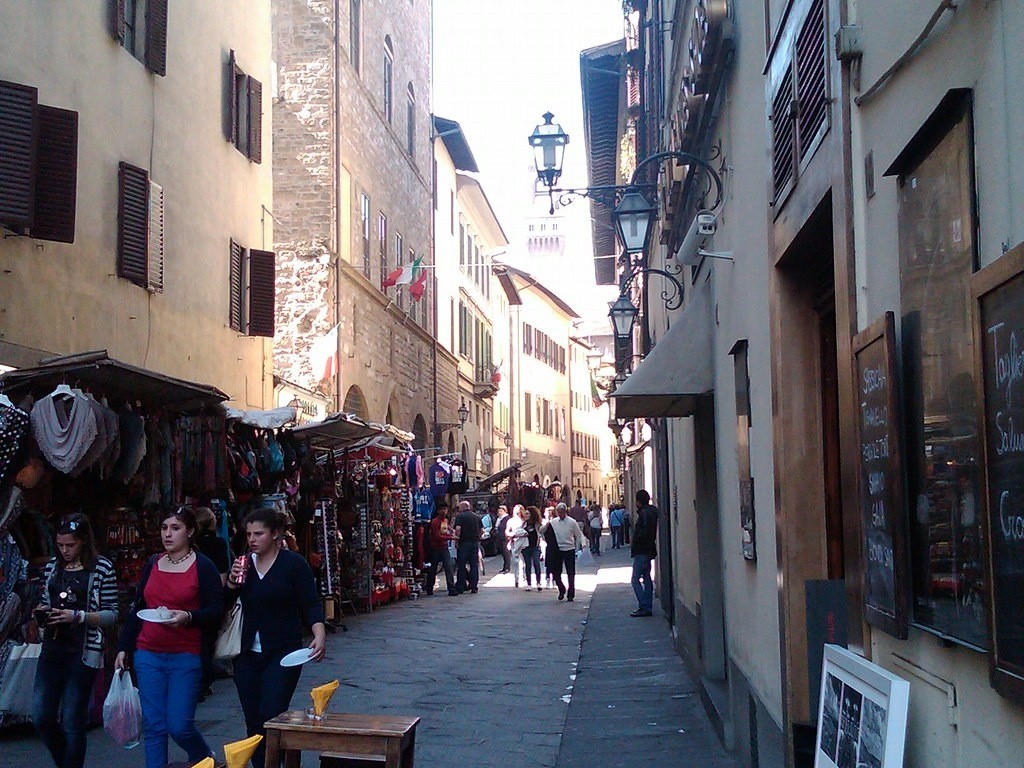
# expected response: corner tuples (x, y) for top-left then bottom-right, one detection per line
(568, 596), (573, 602)
(525, 585), (532, 591)
(448, 591), (458, 596)
(617, 546), (621, 549)
(537, 583), (543, 591)
(558, 587), (566, 600)
(630, 610), (651, 617)
(612, 544), (615, 548)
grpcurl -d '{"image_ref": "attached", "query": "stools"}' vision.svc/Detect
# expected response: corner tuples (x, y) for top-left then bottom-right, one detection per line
(319, 750), (387, 768)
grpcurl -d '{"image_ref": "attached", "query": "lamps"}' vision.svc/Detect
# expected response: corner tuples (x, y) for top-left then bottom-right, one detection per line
(429, 402), (470, 434)
(484, 432), (512, 455)
(612, 354), (645, 391)
(530, 111), (657, 215)
(607, 264), (684, 345)
(573, 464), (589, 477)
(609, 146), (725, 258)
(607, 391), (625, 439)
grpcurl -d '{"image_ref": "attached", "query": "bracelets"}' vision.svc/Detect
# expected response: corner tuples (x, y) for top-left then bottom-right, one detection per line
(185, 611), (190, 625)
(73, 610), (90, 624)
(227, 576), (236, 586)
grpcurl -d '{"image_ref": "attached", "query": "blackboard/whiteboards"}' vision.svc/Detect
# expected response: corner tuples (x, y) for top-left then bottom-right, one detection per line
(970, 241), (1024, 702)
(852, 311), (912, 639)
(728, 340), (757, 561)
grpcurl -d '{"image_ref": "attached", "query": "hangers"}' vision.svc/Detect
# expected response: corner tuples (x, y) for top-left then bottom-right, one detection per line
(0, 379), (145, 420)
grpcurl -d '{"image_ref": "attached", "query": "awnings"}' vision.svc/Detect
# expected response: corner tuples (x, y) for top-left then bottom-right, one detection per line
(612, 279), (715, 418)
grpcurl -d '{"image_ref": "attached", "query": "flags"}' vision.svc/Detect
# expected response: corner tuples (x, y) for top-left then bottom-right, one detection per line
(408, 269), (427, 303)
(383, 254), (423, 286)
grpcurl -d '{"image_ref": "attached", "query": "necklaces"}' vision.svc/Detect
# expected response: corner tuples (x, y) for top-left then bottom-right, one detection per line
(65, 563), (82, 568)
(167, 547), (193, 564)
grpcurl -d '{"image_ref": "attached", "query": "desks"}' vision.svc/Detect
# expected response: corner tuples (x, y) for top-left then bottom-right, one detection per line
(263, 710), (421, 768)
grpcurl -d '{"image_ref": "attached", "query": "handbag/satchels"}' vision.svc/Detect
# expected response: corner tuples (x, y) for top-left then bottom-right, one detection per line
(102, 668), (142, 750)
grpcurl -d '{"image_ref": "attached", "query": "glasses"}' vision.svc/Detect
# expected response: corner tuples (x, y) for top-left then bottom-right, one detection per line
(176, 506), (185, 515)
(53, 519), (80, 531)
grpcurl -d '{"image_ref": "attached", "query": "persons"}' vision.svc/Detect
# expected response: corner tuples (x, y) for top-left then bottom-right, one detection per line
(426, 498), (629, 603)
(191, 508), (229, 703)
(630, 490), (659, 616)
(223, 509), (326, 768)
(114, 507), (224, 768)
(35, 513), (120, 768)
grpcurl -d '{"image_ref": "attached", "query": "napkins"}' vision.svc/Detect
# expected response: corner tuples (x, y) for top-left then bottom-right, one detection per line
(310, 679), (340, 716)
(190, 757), (215, 768)
(224, 734), (263, 768)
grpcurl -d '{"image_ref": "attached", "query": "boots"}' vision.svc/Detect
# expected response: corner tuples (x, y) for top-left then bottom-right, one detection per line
(546, 578), (550, 589)
(552, 579), (556, 588)
